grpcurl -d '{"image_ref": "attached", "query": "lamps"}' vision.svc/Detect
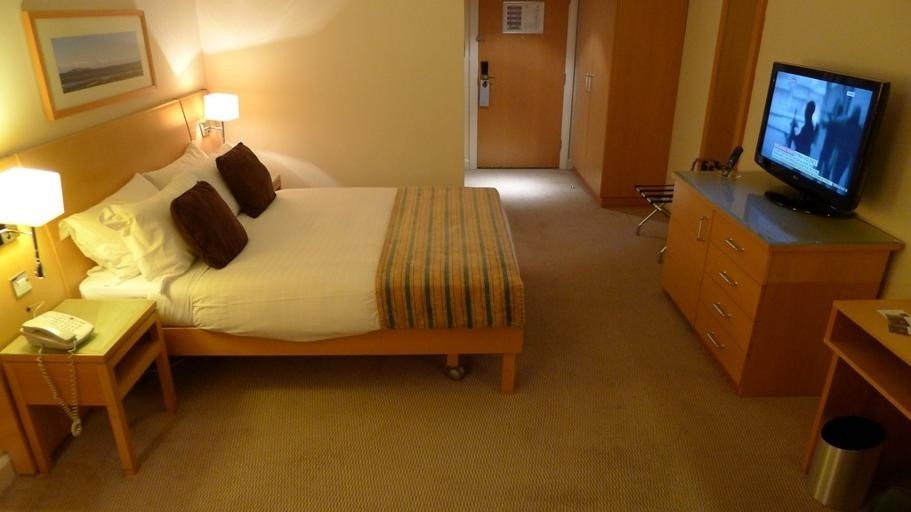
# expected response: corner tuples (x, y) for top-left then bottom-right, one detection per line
(0, 166), (65, 278)
(201, 92), (240, 143)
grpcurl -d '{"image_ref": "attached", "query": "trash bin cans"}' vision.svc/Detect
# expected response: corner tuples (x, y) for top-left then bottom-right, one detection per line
(808, 414), (890, 511)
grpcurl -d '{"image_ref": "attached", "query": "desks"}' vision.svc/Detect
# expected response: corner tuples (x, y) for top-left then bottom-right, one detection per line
(796, 298), (910, 478)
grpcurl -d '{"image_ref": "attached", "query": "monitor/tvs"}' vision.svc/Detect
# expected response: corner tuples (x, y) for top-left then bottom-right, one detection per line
(754, 62), (891, 219)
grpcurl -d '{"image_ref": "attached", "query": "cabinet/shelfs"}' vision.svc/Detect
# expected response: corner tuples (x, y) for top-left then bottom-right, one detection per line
(658, 168), (905, 399)
(565, 0), (690, 209)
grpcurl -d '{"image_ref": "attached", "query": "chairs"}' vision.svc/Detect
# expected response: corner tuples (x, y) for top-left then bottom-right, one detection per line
(633, 145), (746, 264)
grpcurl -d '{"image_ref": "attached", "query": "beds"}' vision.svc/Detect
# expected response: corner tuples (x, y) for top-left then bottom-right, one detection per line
(0, 89), (526, 480)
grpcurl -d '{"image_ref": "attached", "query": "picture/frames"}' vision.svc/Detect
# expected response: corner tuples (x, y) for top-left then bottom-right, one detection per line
(22, 9), (159, 121)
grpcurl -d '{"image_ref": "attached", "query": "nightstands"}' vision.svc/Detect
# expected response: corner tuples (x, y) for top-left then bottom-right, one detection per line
(0, 298), (179, 475)
(272, 174), (286, 189)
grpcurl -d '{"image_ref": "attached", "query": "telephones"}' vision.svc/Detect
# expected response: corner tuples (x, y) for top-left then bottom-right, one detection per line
(19, 310), (95, 350)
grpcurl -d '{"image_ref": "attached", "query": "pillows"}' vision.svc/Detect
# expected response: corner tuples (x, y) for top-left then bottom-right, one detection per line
(215, 142), (276, 219)
(58, 173), (161, 279)
(171, 180), (249, 269)
(99, 171), (229, 295)
(143, 141), (209, 189)
(187, 143), (250, 219)
